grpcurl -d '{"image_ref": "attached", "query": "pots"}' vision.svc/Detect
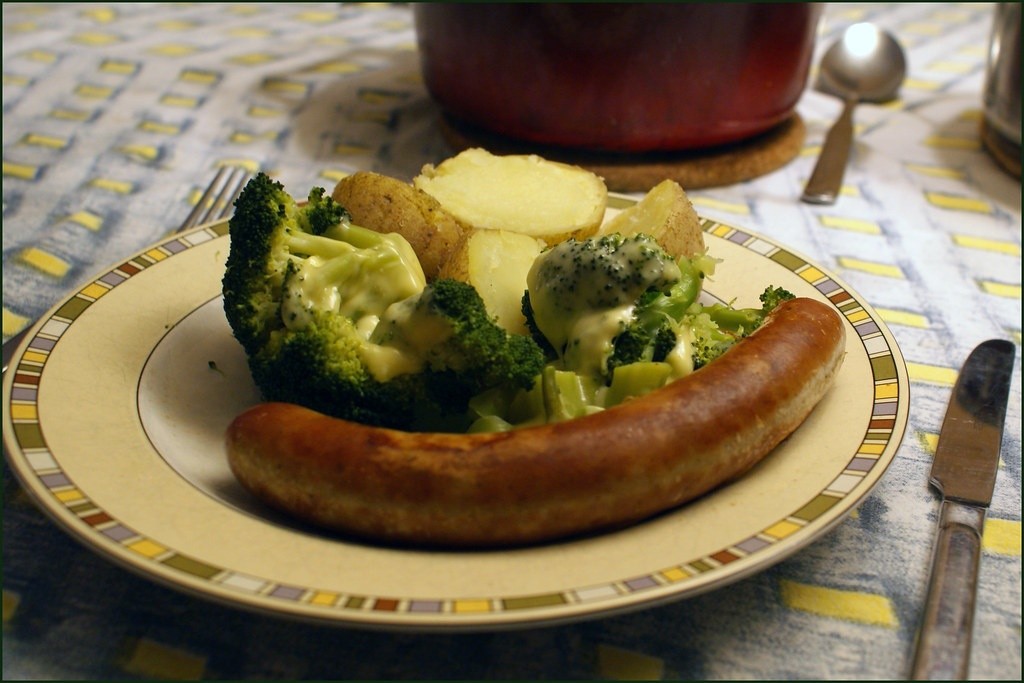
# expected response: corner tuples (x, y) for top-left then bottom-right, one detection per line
(410, 0), (818, 154)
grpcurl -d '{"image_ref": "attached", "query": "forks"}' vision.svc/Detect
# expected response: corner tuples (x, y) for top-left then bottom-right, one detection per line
(0, 163), (254, 379)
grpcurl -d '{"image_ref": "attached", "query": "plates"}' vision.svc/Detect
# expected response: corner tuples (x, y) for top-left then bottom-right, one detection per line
(0, 189), (911, 634)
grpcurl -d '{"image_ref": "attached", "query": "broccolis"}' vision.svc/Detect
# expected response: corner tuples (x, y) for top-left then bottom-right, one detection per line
(220, 173), (800, 431)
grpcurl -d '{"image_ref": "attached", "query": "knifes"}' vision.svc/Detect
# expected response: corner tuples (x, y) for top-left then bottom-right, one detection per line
(911, 338), (1017, 682)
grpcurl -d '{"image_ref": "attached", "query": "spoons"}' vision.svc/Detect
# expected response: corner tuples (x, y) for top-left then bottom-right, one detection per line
(802, 22), (907, 206)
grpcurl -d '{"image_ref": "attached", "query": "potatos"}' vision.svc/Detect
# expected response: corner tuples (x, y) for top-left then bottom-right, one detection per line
(328, 146), (708, 332)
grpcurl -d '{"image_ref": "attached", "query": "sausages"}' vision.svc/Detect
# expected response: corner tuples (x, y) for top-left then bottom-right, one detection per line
(223, 298), (847, 547)
